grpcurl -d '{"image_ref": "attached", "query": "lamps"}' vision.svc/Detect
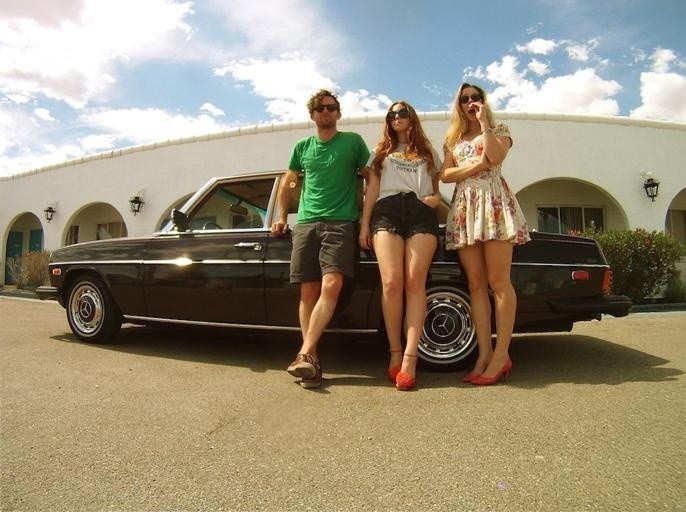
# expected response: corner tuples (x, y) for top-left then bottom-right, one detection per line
(642, 170), (661, 202)
(128, 195), (145, 216)
(43, 207), (56, 223)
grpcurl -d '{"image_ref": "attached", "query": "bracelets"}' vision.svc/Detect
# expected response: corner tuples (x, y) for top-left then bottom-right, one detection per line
(480, 127), (495, 134)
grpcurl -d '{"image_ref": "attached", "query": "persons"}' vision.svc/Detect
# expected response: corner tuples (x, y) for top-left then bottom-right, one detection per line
(269, 90), (371, 388)
(357, 99), (442, 392)
(439, 81), (533, 385)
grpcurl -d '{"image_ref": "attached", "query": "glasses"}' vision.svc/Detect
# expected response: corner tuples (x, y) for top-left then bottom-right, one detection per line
(387, 108), (408, 122)
(460, 94), (480, 103)
(314, 104), (337, 111)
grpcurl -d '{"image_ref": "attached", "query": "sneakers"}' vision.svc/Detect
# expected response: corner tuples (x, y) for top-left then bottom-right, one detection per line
(287, 354), (322, 388)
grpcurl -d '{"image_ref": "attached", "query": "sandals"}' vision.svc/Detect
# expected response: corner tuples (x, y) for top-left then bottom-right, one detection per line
(389, 350), (418, 390)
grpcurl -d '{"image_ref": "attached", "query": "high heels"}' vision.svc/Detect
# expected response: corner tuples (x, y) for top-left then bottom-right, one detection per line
(463, 358), (512, 384)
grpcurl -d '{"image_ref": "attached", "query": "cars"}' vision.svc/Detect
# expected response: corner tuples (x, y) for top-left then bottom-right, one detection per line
(35, 164), (637, 370)
